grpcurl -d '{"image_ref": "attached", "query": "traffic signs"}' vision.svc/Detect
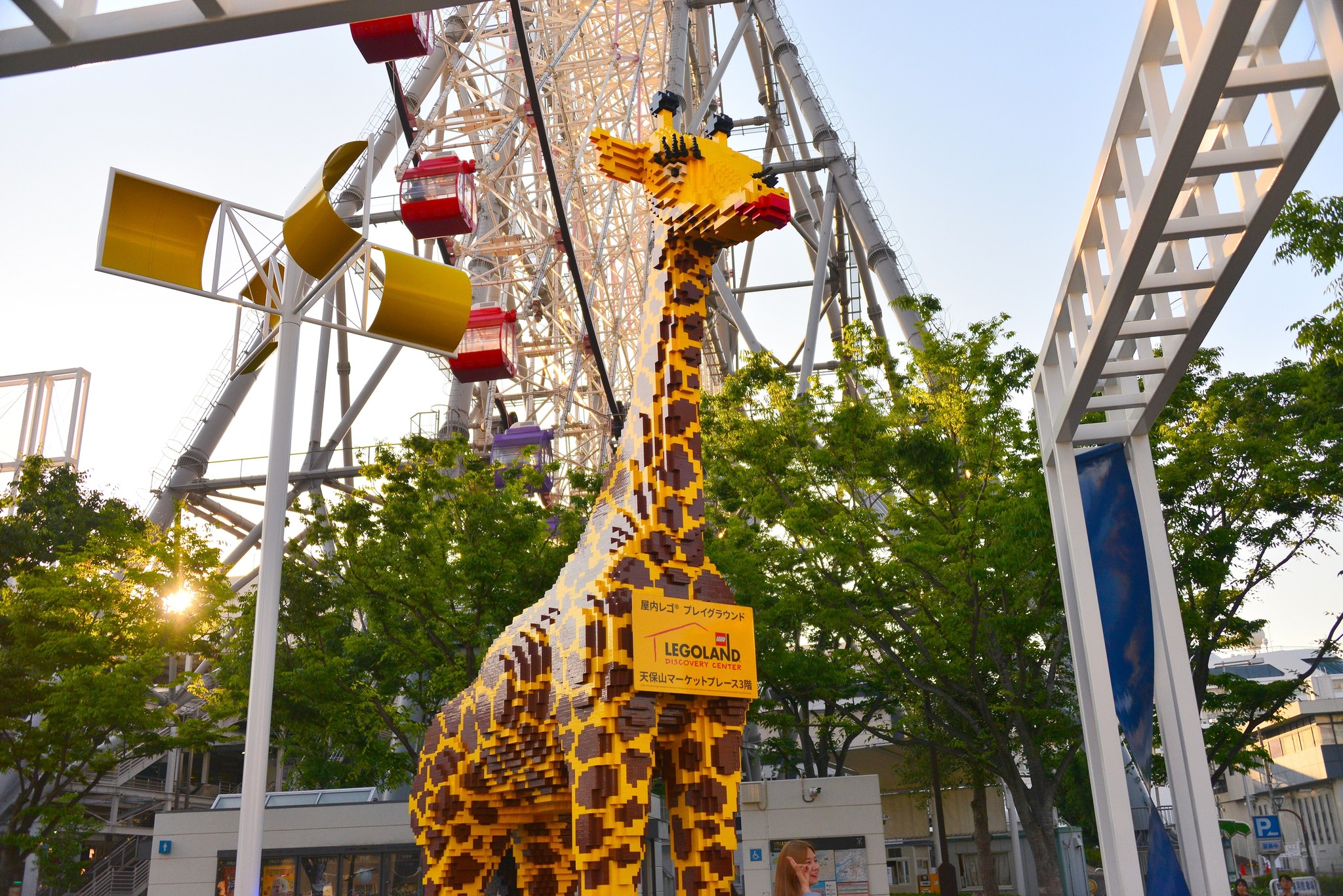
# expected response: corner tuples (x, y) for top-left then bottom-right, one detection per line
(1252, 814), (1286, 855)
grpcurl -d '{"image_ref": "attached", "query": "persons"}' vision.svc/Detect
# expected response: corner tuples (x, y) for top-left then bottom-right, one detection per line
(1233, 878), (1252, 896)
(1277, 875), (1301, 896)
(773, 840), (821, 896)
(401, 182), (427, 203)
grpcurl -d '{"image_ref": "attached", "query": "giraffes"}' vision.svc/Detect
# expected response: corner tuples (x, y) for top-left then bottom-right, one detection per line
(399, 85), (797, 896)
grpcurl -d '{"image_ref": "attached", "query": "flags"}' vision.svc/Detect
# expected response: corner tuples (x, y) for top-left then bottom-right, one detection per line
(1076, 451), (1155, 779)
(1146, 797), (1191, 896)
